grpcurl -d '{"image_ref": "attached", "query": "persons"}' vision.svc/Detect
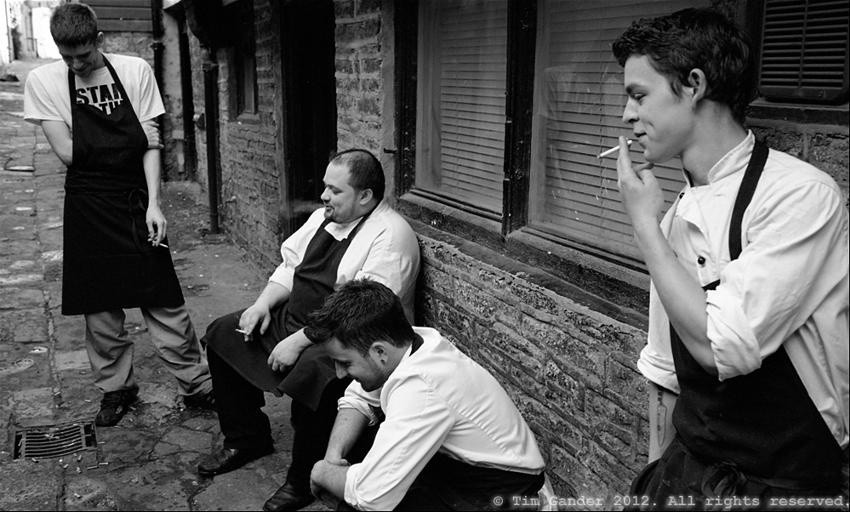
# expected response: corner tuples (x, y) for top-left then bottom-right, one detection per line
(23, 3), (218, 427)
(198, 148), (422, 511)
(611, 7), (849, 512)
(303, 279), (547, 512)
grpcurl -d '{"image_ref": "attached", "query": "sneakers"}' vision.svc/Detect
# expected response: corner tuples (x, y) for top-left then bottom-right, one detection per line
(183, 388), (217, 412)
(94, 381), (140, 428)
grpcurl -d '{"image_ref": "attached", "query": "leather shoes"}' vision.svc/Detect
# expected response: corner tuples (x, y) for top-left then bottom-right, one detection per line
(198, 447), (276, 478)
(262, 480), (317, 512)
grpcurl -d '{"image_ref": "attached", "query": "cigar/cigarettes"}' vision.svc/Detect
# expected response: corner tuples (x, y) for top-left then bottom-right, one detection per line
(235, 329), (253, 336)
(597, 140), (632, 159)
(152, 240), (168, 248)
